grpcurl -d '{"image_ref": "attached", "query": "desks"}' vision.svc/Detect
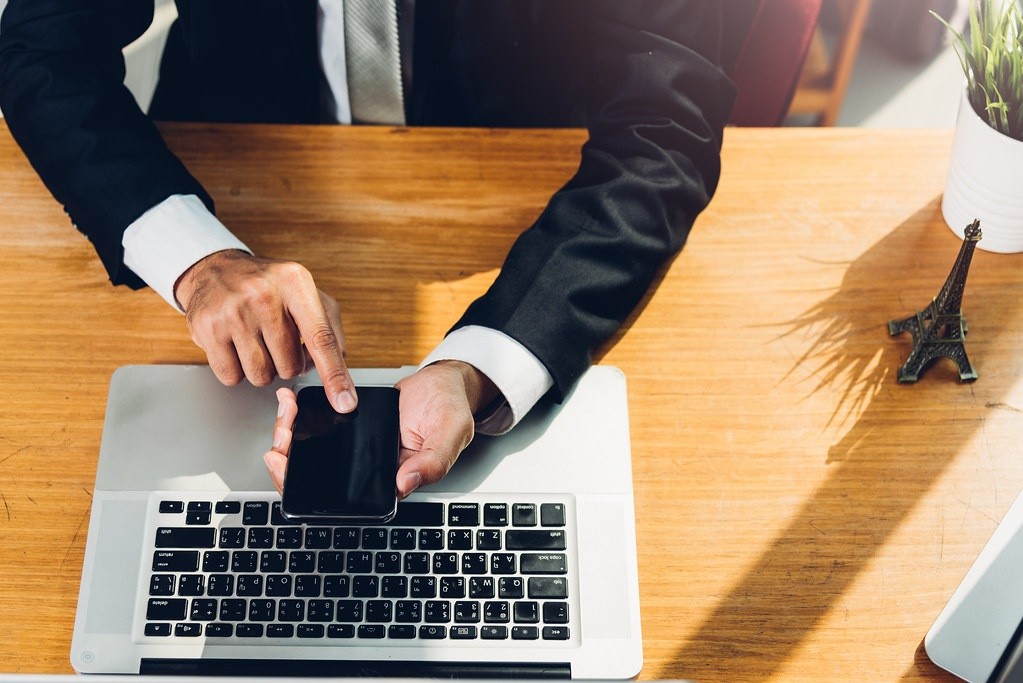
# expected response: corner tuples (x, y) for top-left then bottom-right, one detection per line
(0, 119), (1023, 683)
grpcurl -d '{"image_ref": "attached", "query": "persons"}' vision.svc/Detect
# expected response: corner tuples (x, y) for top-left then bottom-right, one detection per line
(0, 0), (736, 498)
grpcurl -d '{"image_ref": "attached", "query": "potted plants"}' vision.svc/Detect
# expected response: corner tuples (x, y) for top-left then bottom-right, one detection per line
(930, 0), (1023, 253)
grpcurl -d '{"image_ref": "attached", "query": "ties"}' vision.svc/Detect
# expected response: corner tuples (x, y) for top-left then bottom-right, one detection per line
(343, 0), (407, 126)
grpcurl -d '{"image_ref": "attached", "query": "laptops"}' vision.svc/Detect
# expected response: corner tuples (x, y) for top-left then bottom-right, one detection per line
(69, 365), (644, 678)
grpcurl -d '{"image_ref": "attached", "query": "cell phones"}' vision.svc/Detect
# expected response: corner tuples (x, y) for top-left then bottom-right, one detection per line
(281, 387), (401, 524)
